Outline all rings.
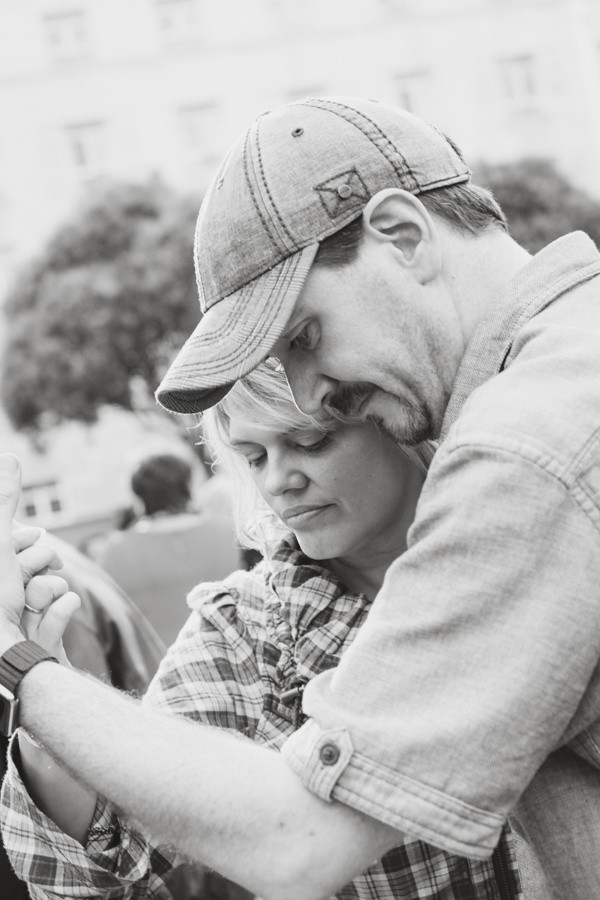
[25,603,43,614]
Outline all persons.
[0,94,600,900]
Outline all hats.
[133,454,192,508]
[153,95,470,411]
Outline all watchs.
[0,640,59,737]
